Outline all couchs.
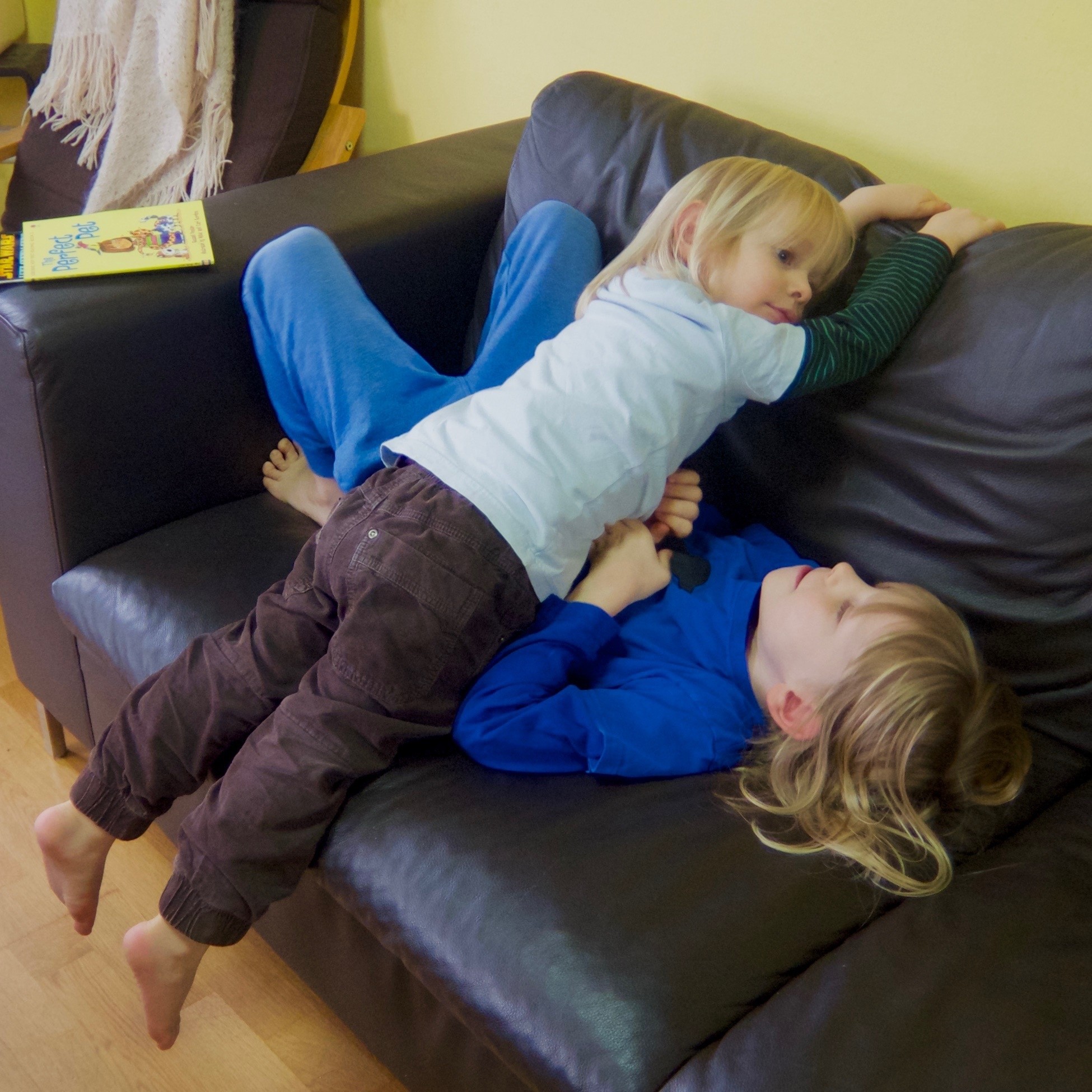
[0,69,1092,1092]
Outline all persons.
[36,160,1008,1048]
[243,200,1033,900]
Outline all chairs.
[0,0,366,233]
